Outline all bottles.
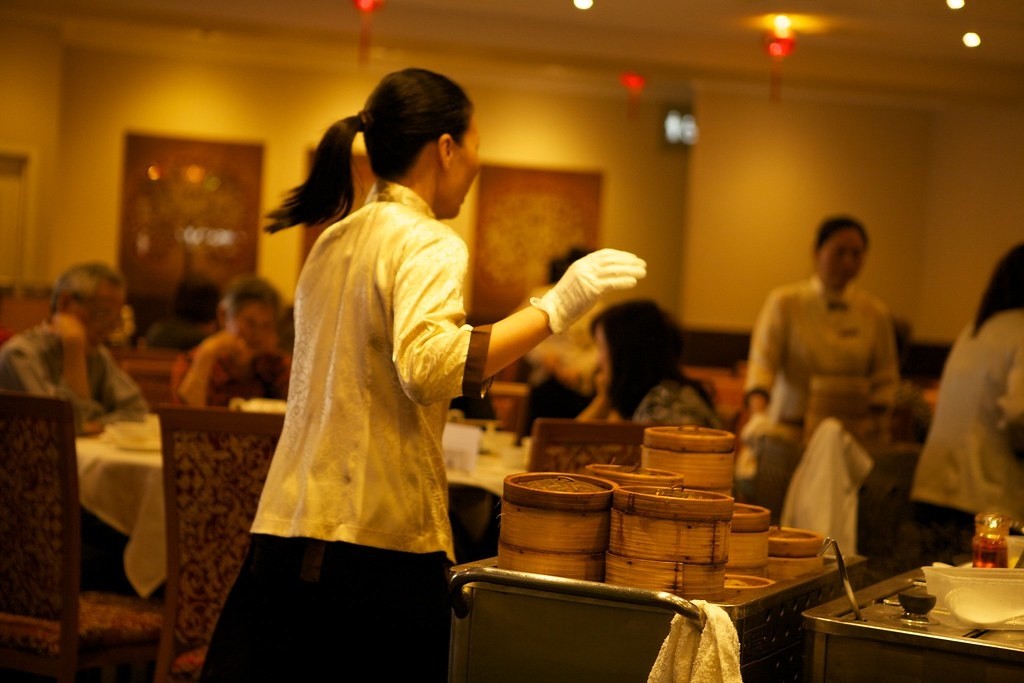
[972,512,1011,568]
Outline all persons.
[193,68,646,683]
[578,297,720,429]
[506,245,608,429]
[0,261,149,439]
[906,242,1024,561]
[174,272,292,408]
[746,217,898,442]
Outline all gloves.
[530,248,646,335]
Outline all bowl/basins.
[441,421,479,480]
[921,564,1024,631]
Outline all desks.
[77,433,529,600]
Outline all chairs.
[0,349,663,683]
